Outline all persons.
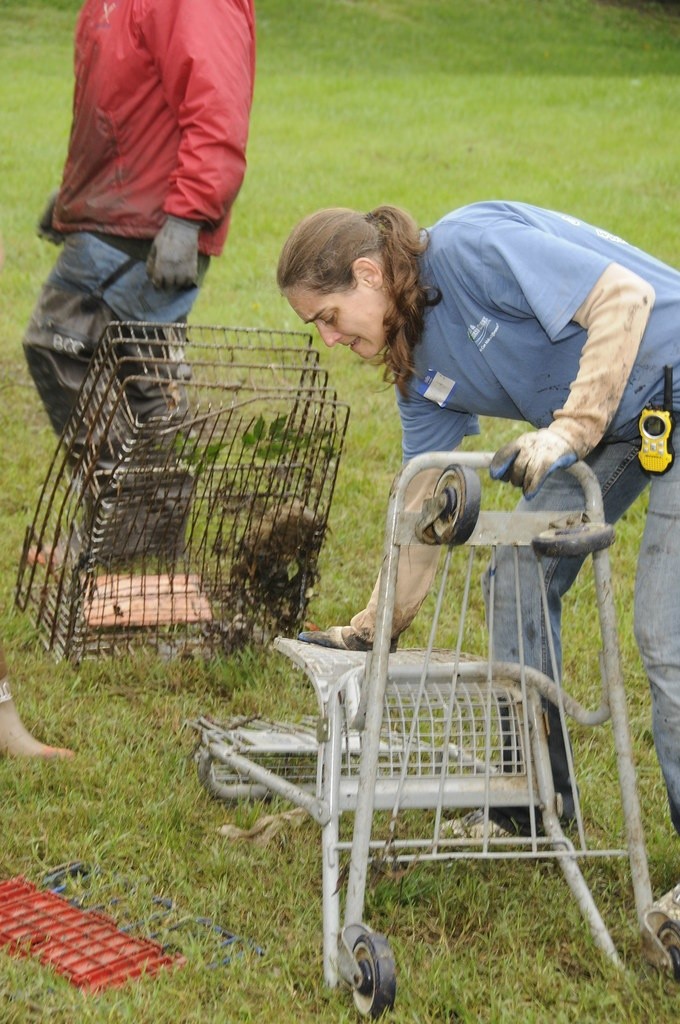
[21,0,257,557]
[276,199,680,920]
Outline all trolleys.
[186,451,680,1022]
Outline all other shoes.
[439,810,513,837]
[654,883,680,919]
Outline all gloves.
[488,428,578,500]
[145,215,204,291]
[298,627,351,651]
[37,193,63,244]
